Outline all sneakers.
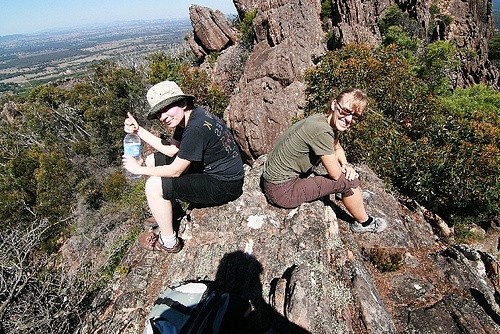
[350,215,388,233]
[337,192,369,200]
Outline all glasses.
[337,102,360,120]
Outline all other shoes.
[139,233,181,252]
[145,215,156,226]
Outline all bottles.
[123,126,141,179]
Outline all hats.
[146,81,196,119]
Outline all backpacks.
[140,279,221,334]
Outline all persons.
[261,88,386,234]
[121,81,243,253]
